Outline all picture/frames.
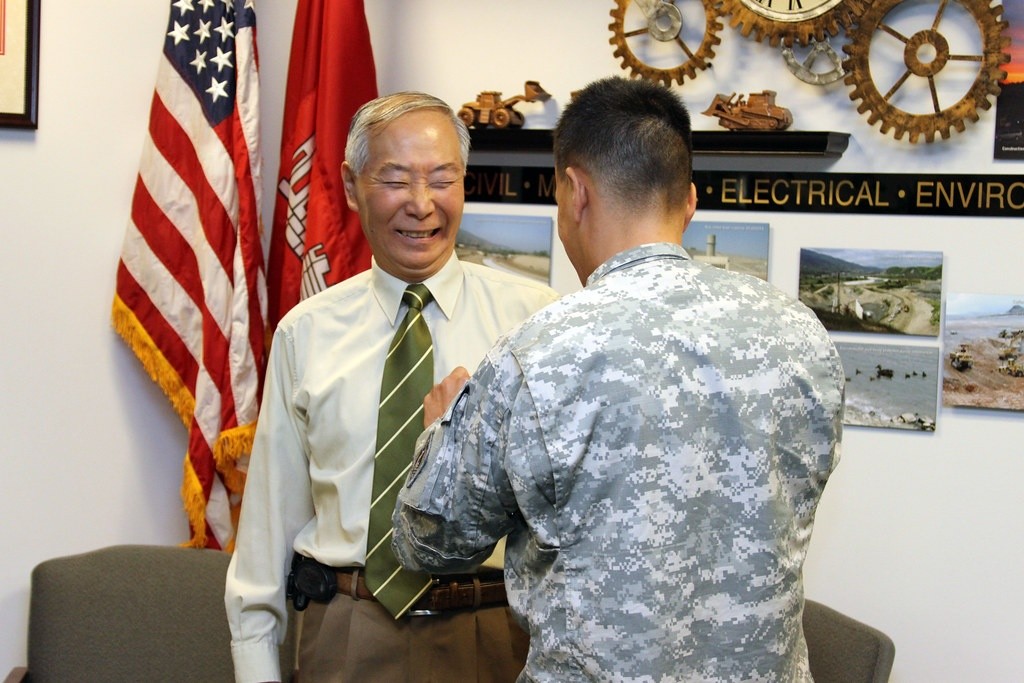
[0,0,41,131]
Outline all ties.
[364,283,433,617]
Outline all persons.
[224,90,561,683]
[389,76,847,683]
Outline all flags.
[111,0,273,552]
[265,0,378,334]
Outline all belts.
[335,573,508,618]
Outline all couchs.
[3,542,896,683]
